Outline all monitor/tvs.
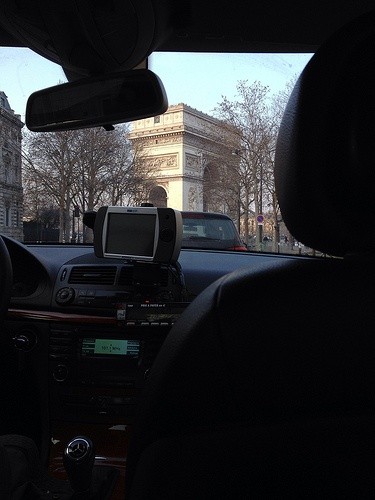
[94,206,183,264]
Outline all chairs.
[126,38,374,499]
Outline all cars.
[179,209,249,252]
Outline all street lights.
[231,148,276,251]
[51,141,120,243]
